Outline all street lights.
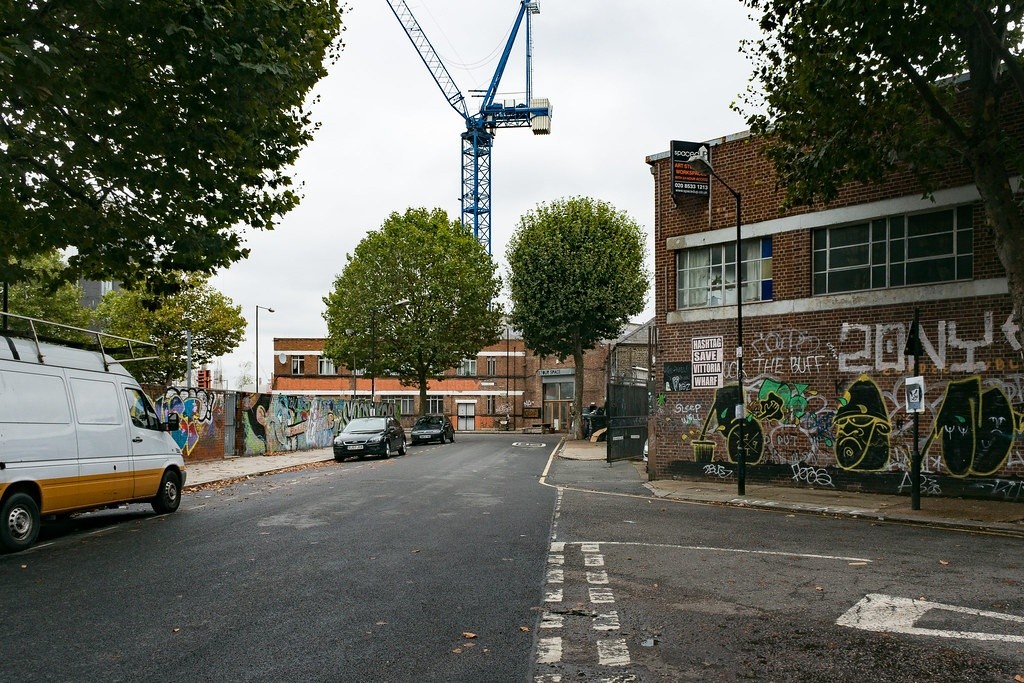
[255,305,275,394]
[686,154,748,500]
[502,324,510,423]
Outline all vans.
[0,312,187,551]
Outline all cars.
[333,417,407,462]
[410,413,455,445]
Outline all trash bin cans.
[580,413,590,439]
[588,414,605,441]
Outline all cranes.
[389,0,552,260]
